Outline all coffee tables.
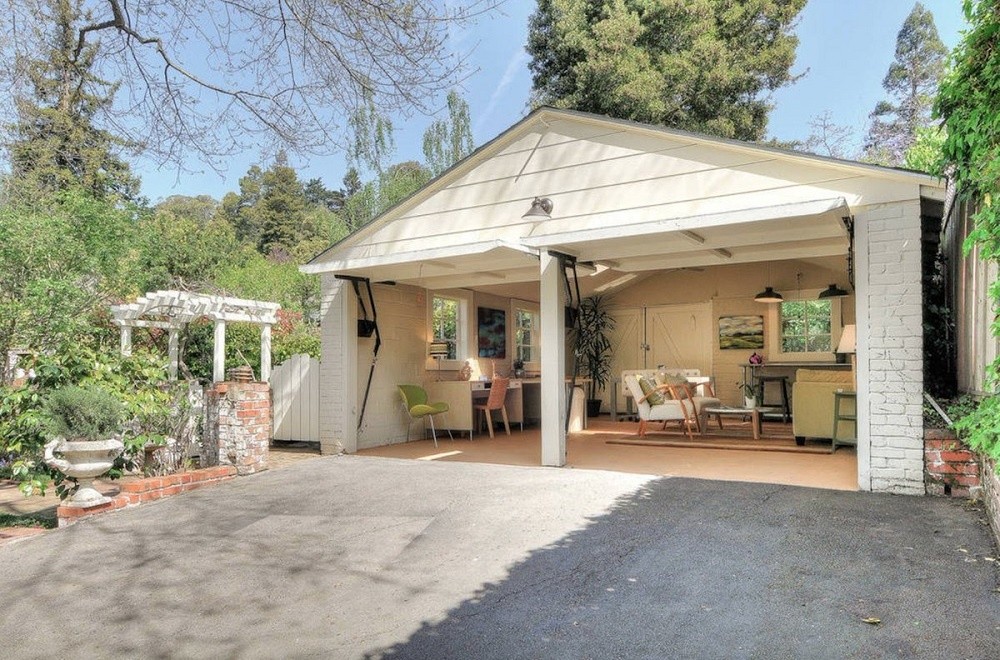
[831,391,857,454]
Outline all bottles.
[461,361,470,380]
[749,351,762,364]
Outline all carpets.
[604,424,841,454]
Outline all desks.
[738,362,853,422]
[423,377,593,442]
[700,406,772,440]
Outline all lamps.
[521,196,553,221]
[836,325,857,391]
[429,343,449,382]
[754,262,785,303]
[817,257,848,298]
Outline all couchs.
[792,368,853,445]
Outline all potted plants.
[566,294,617,417]
[36,381,128,510]
[513,359,524,378]
[738,382,763,408]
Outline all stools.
[753,375,791,425]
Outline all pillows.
[639,373,694,407]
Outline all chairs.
[471,376,510,439]
[396,384,454,448]
[624,372,723,442]
